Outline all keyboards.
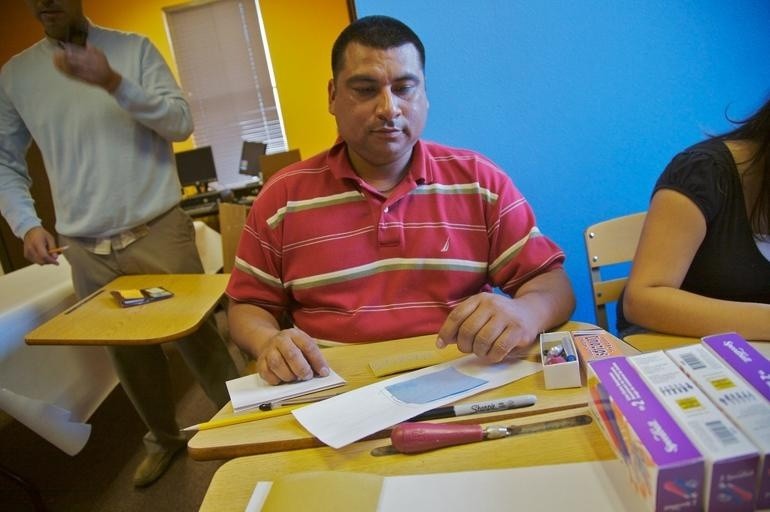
[183,201,216,214]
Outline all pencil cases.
[573,332,624,378]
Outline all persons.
[223,13,578,387]
[0,0,239,489]
[620,97,770,342]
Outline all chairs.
[0,142,304,512]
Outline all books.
[224,363,347,414]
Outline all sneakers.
[132,426,188,487]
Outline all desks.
[187,211,766,511]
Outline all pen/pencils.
[405,395,537,423]
[47,245,69,254]
[179,392,345,432]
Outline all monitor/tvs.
[174,145,218,194]
[240,141,267,187]
